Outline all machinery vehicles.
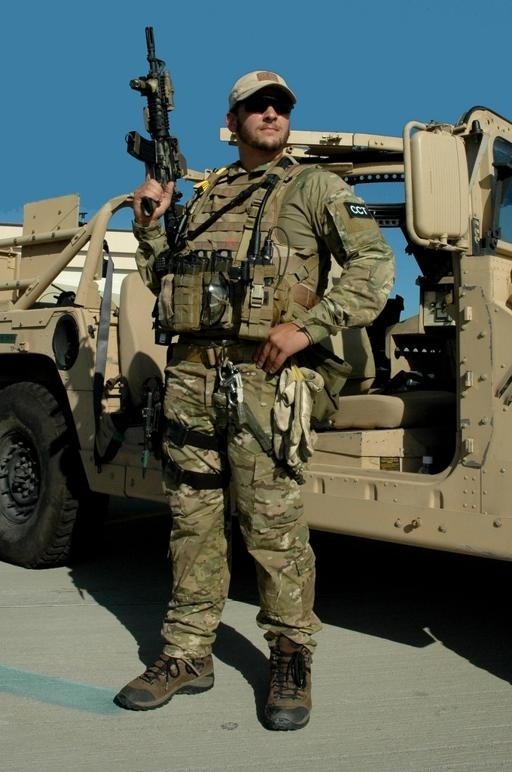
[1,106,511,678]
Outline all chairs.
[116,271,172,415]
[319,270,457,430]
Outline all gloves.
[272,366,324,468]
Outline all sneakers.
[112,651,215,713]
[262,635,314,731]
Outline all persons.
[114,70,394,731]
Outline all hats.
[227,71,299,111]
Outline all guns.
[141,389,153,478]
[127,25,181,252]
